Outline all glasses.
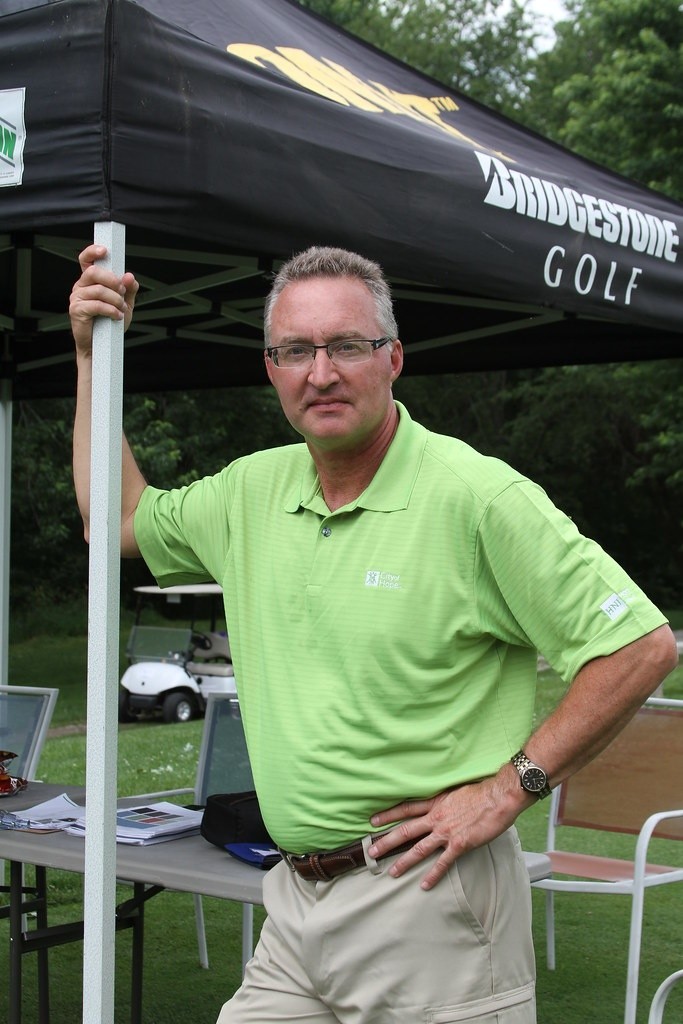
[263,337,390,369]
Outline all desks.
[0,797,552,1024]
[0,782,86,1024]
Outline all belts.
[285,827,435,882]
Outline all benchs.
[185,635,234,677]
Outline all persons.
[62,245,676,1024]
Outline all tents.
[0,1,683,1024]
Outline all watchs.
[510,751,552,801]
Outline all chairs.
[0,685,59,933]
[116,691,255,983]
[530,698,683,1024]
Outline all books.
[65,800,204,846]
